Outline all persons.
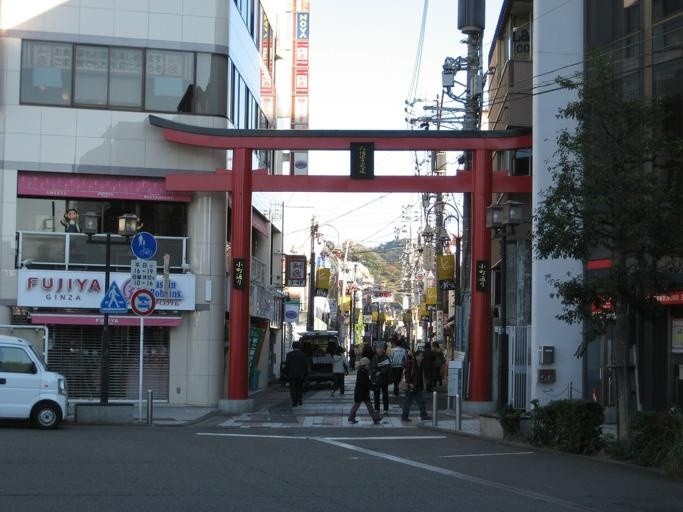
[286,342,306,406]
[332,351,349,394]
[346,334,449,424]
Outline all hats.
[360,357,370,365]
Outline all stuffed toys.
[60,209,80,233]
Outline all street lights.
[422,201,464,351]
[280,291,291,364]
[82,212,144,406]
[348,283,361,349]
[486,201,527,418]
[309,225,343,331]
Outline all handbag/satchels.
[398,375,408,391]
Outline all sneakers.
[292,401,302,406]
[421,416,432,420]
[374,410,388,423]
[348,421,358,424]
[402,419,412,422]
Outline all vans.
[0,335,69,430]
[296,330,342,388]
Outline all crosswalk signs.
[100,280,128,315]
